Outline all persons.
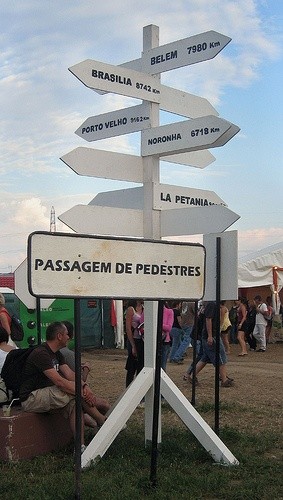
[0,290,282,429]
[22,321,129,455]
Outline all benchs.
[0,405,66,462]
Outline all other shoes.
[188,377,201,387]
[221,378,235,388]
[183,375,192,381]
[249,348,256,351]
[255,348,266,352]
[219,376,233,381]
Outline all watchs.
[82,383,88,389]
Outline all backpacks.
[2,311,25,342]
[259,302,273,320]
[0,344,38,400]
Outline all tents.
[238,242,283,315]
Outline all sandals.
[238,352,248,357]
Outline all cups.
[3,405,11,417]
